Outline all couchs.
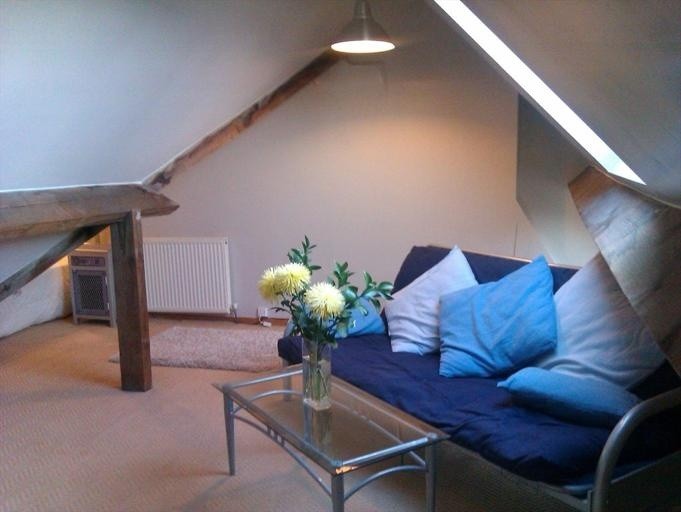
[275,245,681,511]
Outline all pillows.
[438,254,558,379]
[534,251,665,392]
[494,365,662,425]
[385,245,480,355]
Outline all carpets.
[105,324,294,374]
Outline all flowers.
[256,235,398,396]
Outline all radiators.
[140,235,238,317]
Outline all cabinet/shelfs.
[68,245,114,325]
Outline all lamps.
[329,0,397,55]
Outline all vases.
[300,335,334,411]
